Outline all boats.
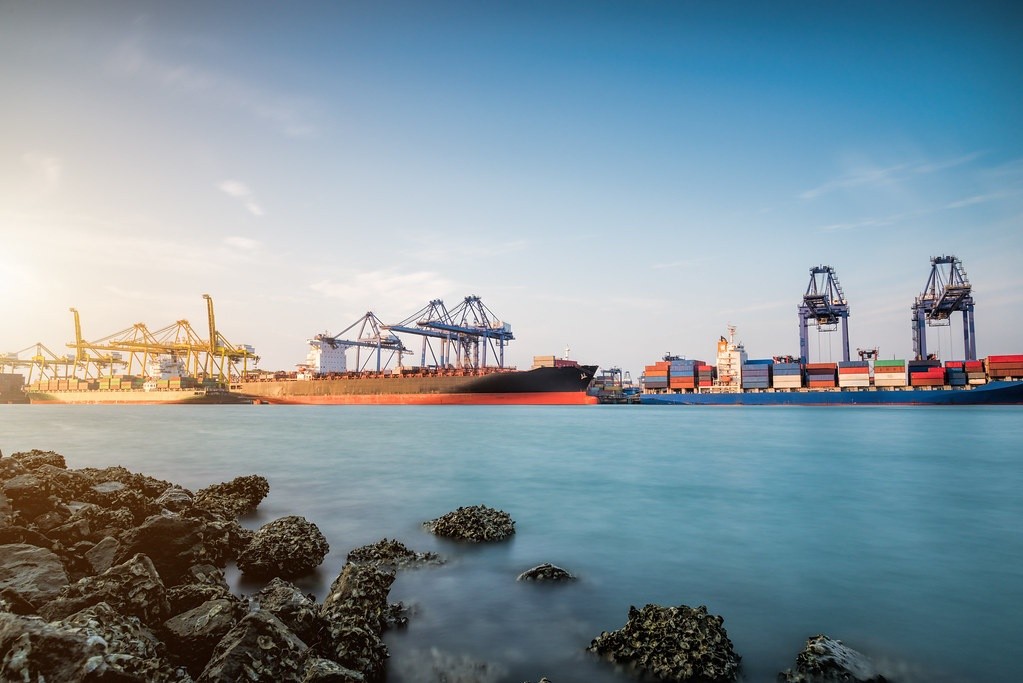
[587,347,1023,404]
[230,364,601,405]
[0,351,239,405]
